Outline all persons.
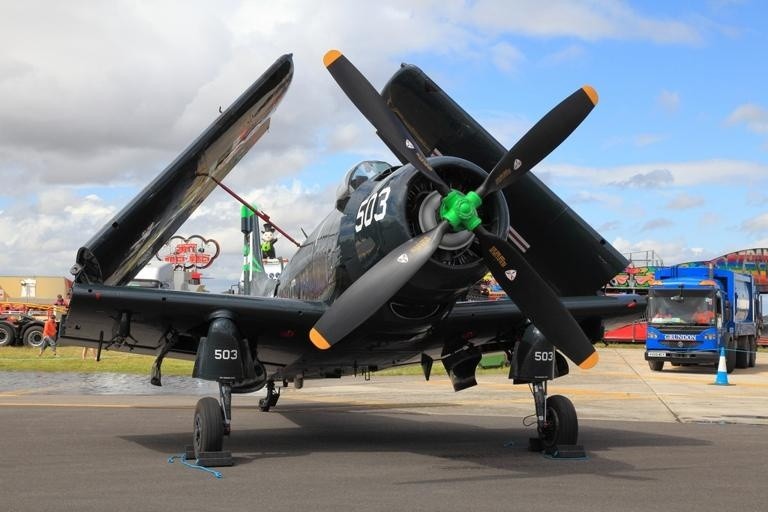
[691,303,714,325]
[654,305,672,318]
[38,314,58,357]
[27,307,33,319]
[54,295,65,306]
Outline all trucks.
[645,267,758,374]
[0,302,69,348]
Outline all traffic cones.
[715,347,729,385]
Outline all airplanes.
[59,49,648,459]
